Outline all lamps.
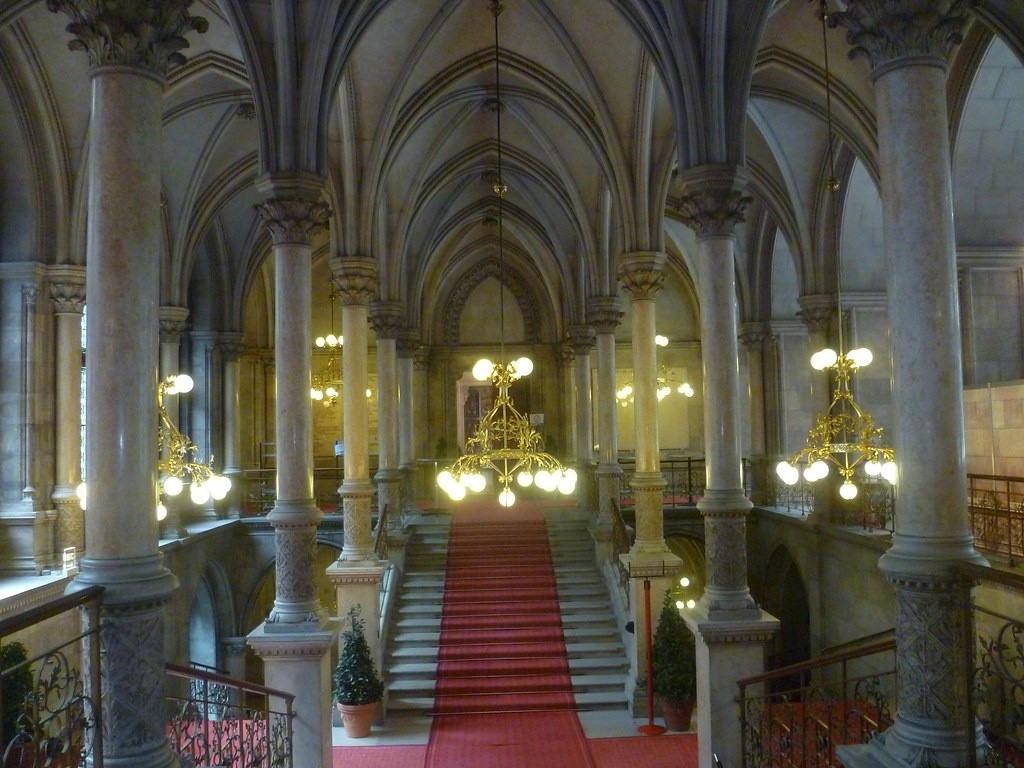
[617,334,694,403]
[437,0,579,507]
[310,272,372,407]
[76,374,231,522]
[775,0,899,500]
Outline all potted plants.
[0,641,44,768]
[635,587,698,731]
[333,604,385,738]
